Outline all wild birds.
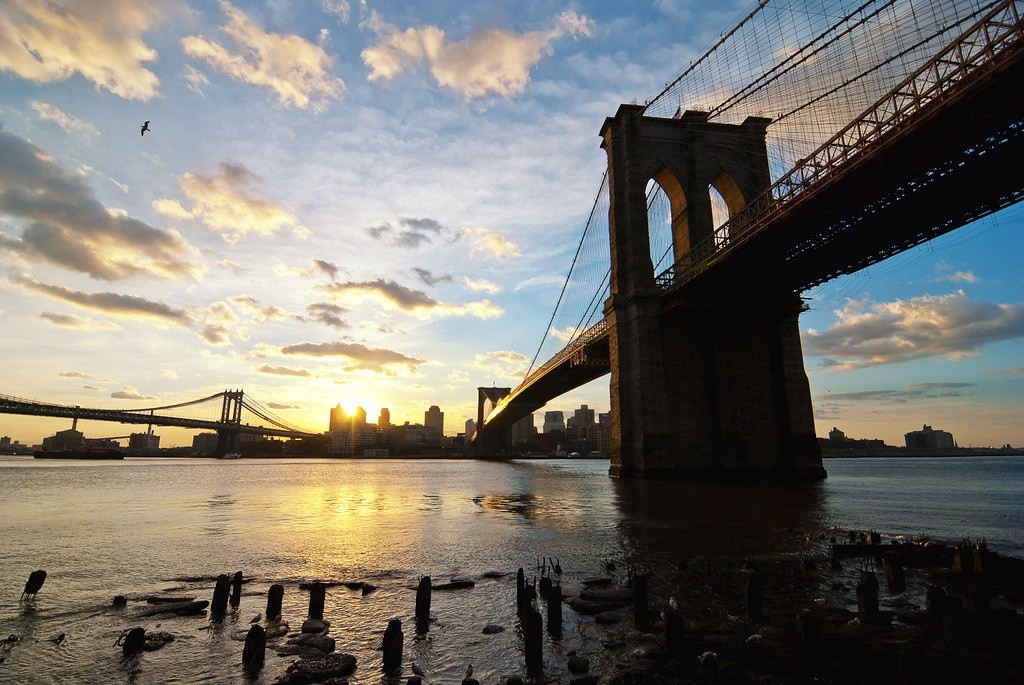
[465,664,475,680]
[669,597,679,609]
[412,661,427,678]
[44,633,65,646]
[140,121,150,136]
[247,613,263,626]
